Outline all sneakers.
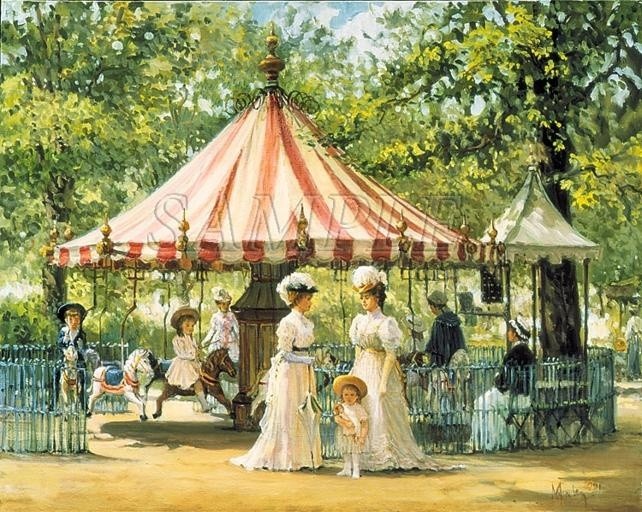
[336,470,352,477]
[351,469,360,480]
[201,404,216,414]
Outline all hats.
[333,374,368,397]
[406,314,427,333]
[508,312,532,342]
[213,290,232,303]
[171,308,200,329]
[57,303,86,323]
[276,272,320,305]
[426,290,448,306]
[351,266,388,294]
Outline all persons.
[470,313,537,451]
[47,299,96,418]
[331,375,370,478]
[200,287,240,418]
[163,304,217,414]
[228,267,326,472]
[619,304,642,378]
[333,404,359,443]
[424,288,467,369]
[349,263,467,473]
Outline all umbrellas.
[483,166,601,360]
